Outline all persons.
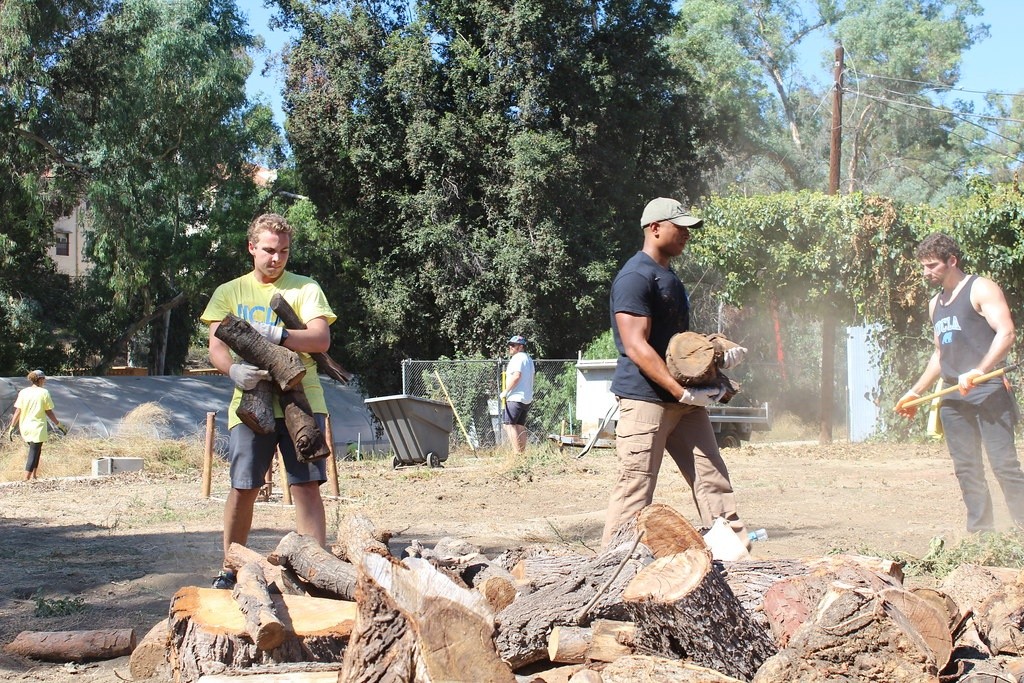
[599,196,748,564]
[893,234,1024,534]
[8,370,68,482]
[499,335,536,454]
[199,214,338,592]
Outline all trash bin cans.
[364,394,453,470]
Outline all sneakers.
[211,570,237,590]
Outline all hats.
[507,336,525,345]
[33,370,45,381]
[641,197,704,229]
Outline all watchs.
[278,328,289,347]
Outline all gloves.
[500,389,509,400]
[720,347,748,369]
[56,421,68,435]
[249,321,283,345]
[679,383,726,406]
[229,364,262,390]
[8,426,15,442]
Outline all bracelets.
[907,390,921,400]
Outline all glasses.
[510,344,518,347]
